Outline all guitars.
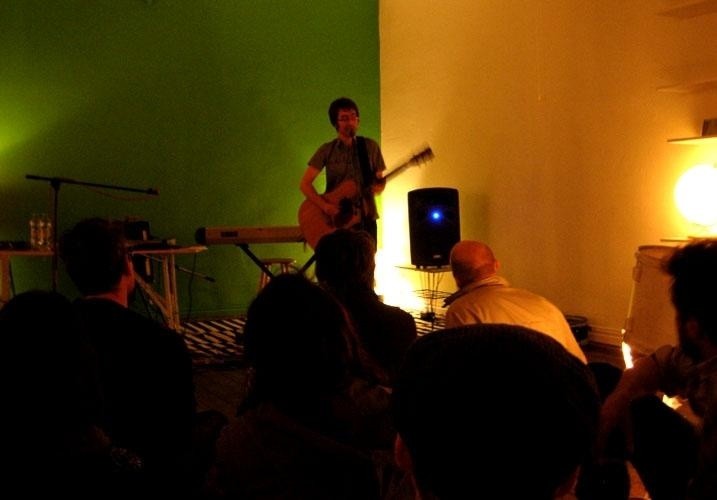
[298,144,435,249]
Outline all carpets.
[179,313,444,370]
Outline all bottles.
[29,211,54,252]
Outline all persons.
[224,227,716,499]
[2,218,222,499]
[300,98,386,290]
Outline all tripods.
[128,246,216,328]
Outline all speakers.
[408,187,460,269]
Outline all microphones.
[349,128,358,154]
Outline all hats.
[397,324,599,492]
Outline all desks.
[397,264,454,321]
[1,240,206,334]
